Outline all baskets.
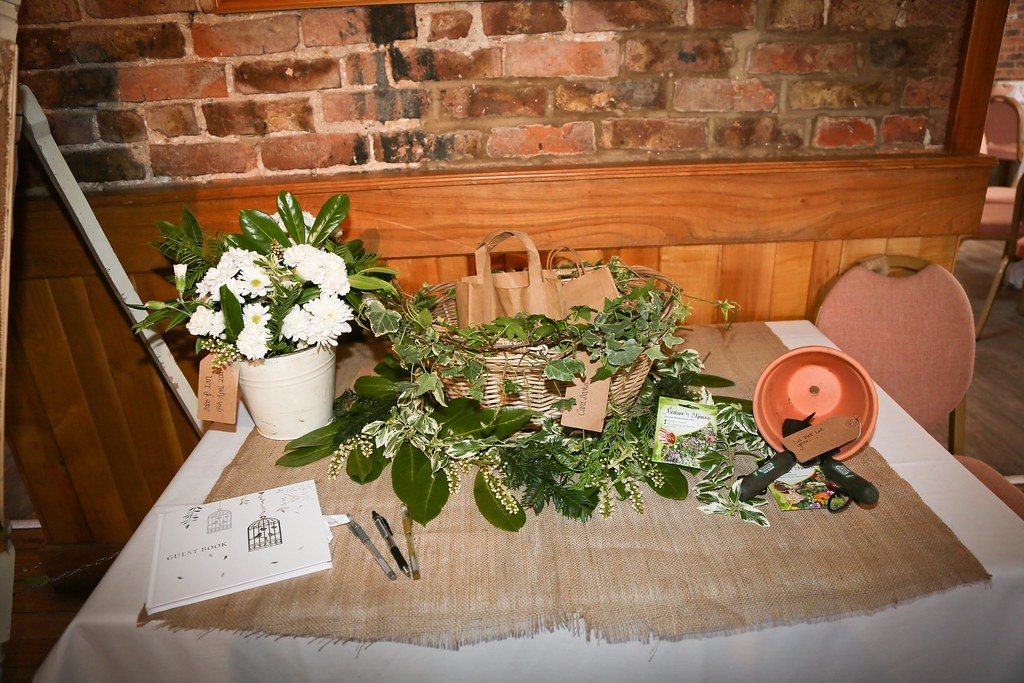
[402,268,681,426]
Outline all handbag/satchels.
[455,231,624,338]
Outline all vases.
[234,340,338,443]
[751,348,878,462]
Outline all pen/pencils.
[372,510,411,578]
[401,503,421,580]
[346,514,398,580]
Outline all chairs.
[815,94,1024,521]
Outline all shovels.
[738,411,881,507]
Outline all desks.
[29,325,1024,683]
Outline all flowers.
[119,189,404,374]
[274,255,771,531]
